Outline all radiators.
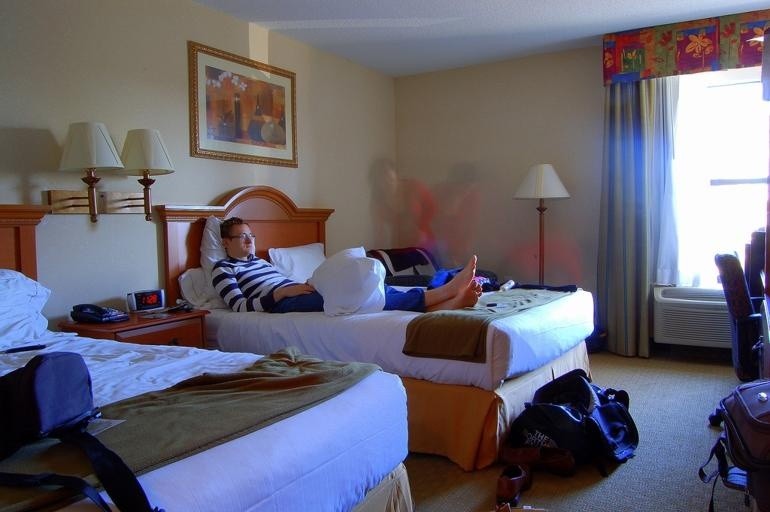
[653,283,732,349]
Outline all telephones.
[70,304,128,323]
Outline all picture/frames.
[187,39,299,169]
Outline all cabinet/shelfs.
[758,270,770,380]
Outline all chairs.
[715,253,764,383]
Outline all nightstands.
[56,307,212,350]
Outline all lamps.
[48,122,175,223]
[512,163,572,286]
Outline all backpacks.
[428,267,500,293]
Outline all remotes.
[7,344,46,353]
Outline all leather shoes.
[496,462,538,506]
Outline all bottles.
[498,280,516,292]
[234,93,285,143]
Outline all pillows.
[177,266,228,311]
[199,215,256,300]
[307,246,387,316]
[267,242,326,283]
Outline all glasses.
[223,233,255,240]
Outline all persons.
[209,215,482,314]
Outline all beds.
[154,185,595,473]
[0,202,417,512]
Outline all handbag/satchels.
[503,368,639,470]
[1,350,164,512]
[719,377,770,472]
